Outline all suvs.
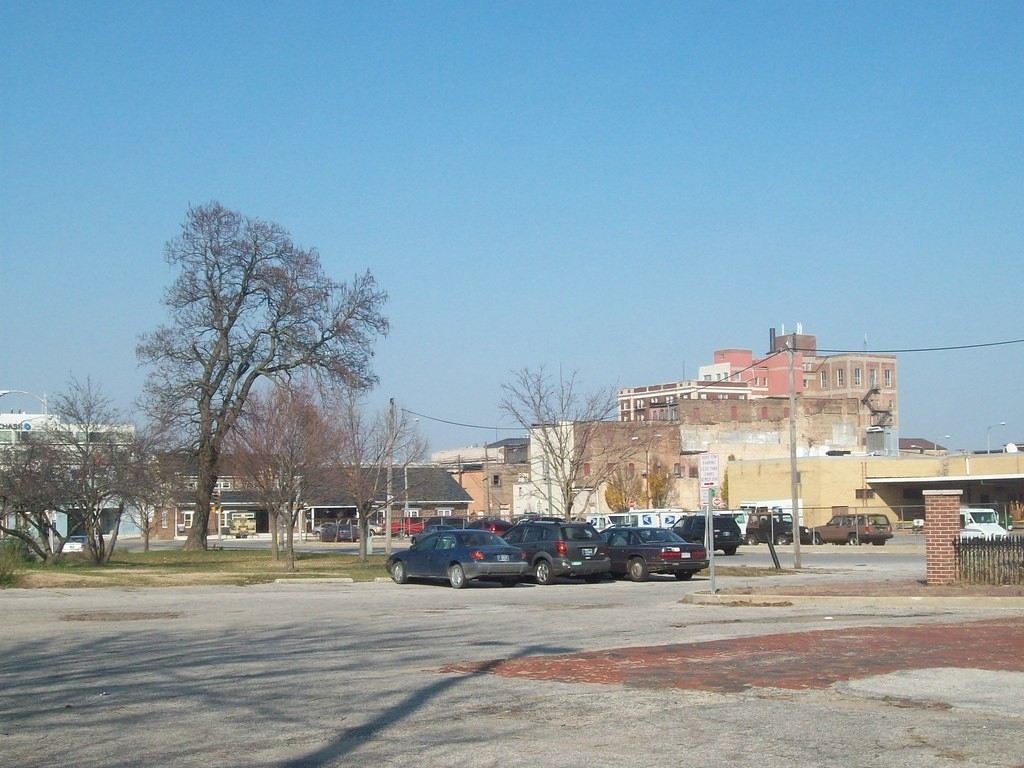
[962,508,1014,540]
[746,512,809,545]
[667,516,743,556]
[502,518,612,586]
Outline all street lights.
[934,436,949,456]
[0,390,49,430]
[987,422,1006,454]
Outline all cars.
[599,524,710,582]
[339,517,384,538]
[312,523,335,541]
[237,531,259,538]
[321,524,357,542]
[384,516,428,537]
[63,536,99,553]
[385,530,529,589]
[467,520,513,537]
[809,514,893,545]
[410,524,457,545]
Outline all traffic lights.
[214,494,220,505]
